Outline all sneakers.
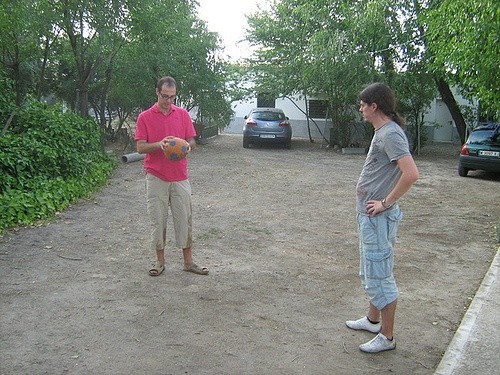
[345,315,382,334]
[358,332,397,353]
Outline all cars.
[457,122,500,178]
[241,108,293,148]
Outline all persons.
[344,82,420,353]
[134,76,210,276]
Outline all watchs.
[380,197,392,209]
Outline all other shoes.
[149,262,164,276]
[182,262,209,275]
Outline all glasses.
[159,91,177,99]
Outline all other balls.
[164,137,188,160]
